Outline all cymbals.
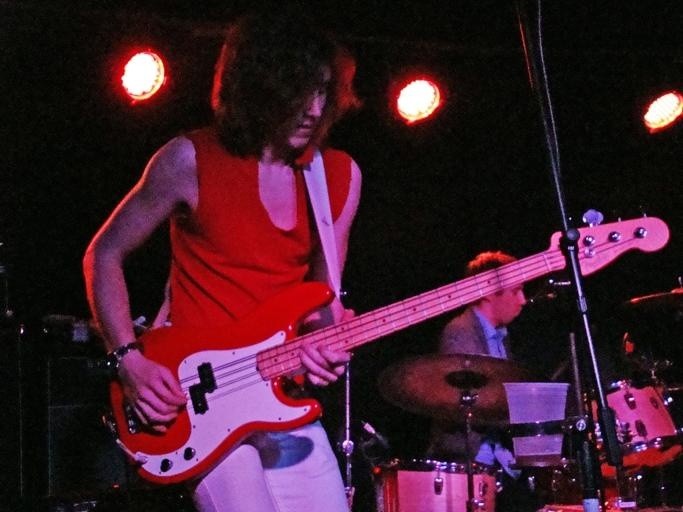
[374,351,540,419]
[620,288,682,306]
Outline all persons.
[426,251,528,464]
[83,12,362,512]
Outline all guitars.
[108,206,670,485]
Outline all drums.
[368,460,502,510]
[581,376,682,479]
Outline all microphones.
[362,423,390,449]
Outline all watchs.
[105,341,144,373]
